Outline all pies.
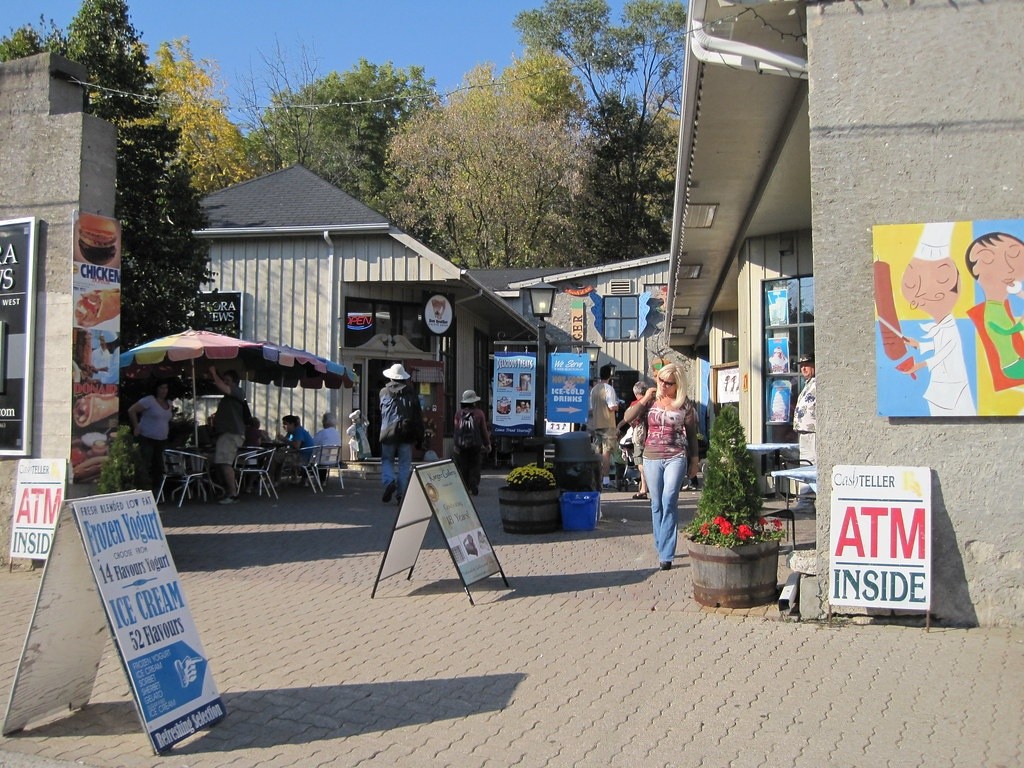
[74,287,121,327]
[74,394,119,427]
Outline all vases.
[498,486,560,535]
[685,538,779,609]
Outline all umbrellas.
[119,324,266,447]
[234,337,362,437]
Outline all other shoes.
[633,492,648,499]
[215,491,240,505]
[321,481,326,487]
[395,495,404,506]
[659,561,672,570]
[471,484,479,496]
[302,481,309,488]
[381,481,398,503]
[602,483,618,491]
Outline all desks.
[176,447,215,497]
[771,465,817,542]
[260,443,288,460]
[744,443,800,500]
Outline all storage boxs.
[558,491,601,531]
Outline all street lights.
[522,275,559,475]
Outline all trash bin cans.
[554,431,602,520]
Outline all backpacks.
[454,408,483,450]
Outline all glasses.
[658,376,676,386]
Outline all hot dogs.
[73,457,111,483]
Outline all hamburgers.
[78,215,117,248]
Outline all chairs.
[758,455,813,550]
[156,444,344,507]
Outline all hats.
[459,390,481,404]
[382,363,411,380]
[796,353,814,364]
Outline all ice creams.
[771,391,786,422]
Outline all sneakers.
[788,502,816,514]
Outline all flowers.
[505,462,556,494]
[679,402,790,549]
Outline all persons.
[309,411,342,488]
[453,389,492,496]
[127,376,174,506]
[589,364,625,491]
[624,363,700,572]
[617,381,655,500]
[203,364,271,506]
[73,327,119,385]
[346,409,372,463]
[380,363,424,506]
[259,414,315,488]
[789,353,818,513]
